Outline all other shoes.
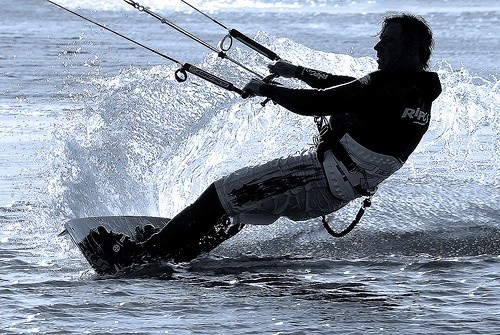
[137,223,172,256]
[93,226,141,265]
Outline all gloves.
[241,78,274,100]
[268,62,298,79]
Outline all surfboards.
[58,215,172,275]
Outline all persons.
[88,10,446,269]
